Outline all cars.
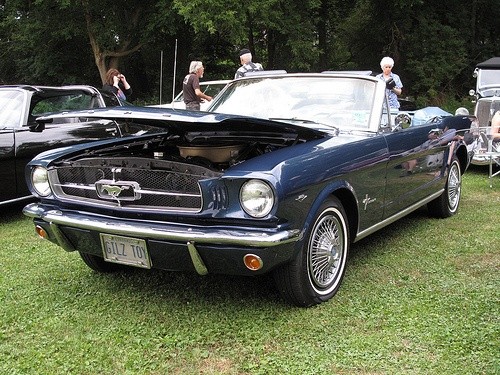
[455,56,500,166]
[0,82,142,213]
[143,79,236,115]
[20,71,481,307]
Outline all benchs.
[31,110,81,123]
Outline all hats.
[238,49,251,56]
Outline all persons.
[489,110,500,152]
[234,49,264,79]
[100,68,133,104]
[183,61,212,111]
[374,56,403,109]
[455,106,484,154]
